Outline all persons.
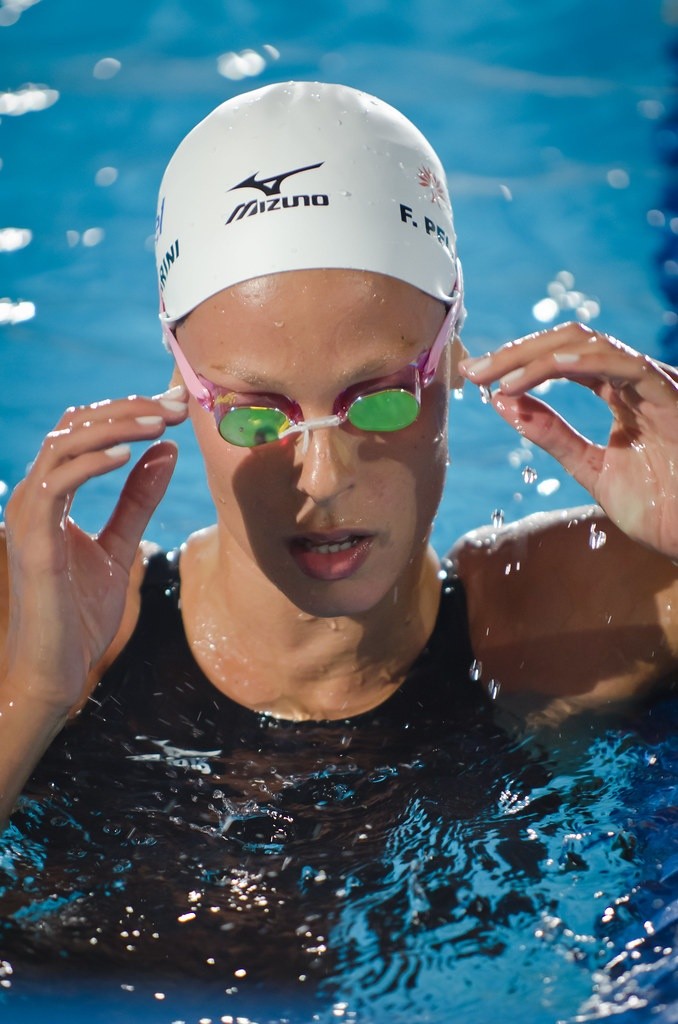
[0,79,678,818]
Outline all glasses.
[195,362,421,447]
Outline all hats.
[152,81,460,331]
